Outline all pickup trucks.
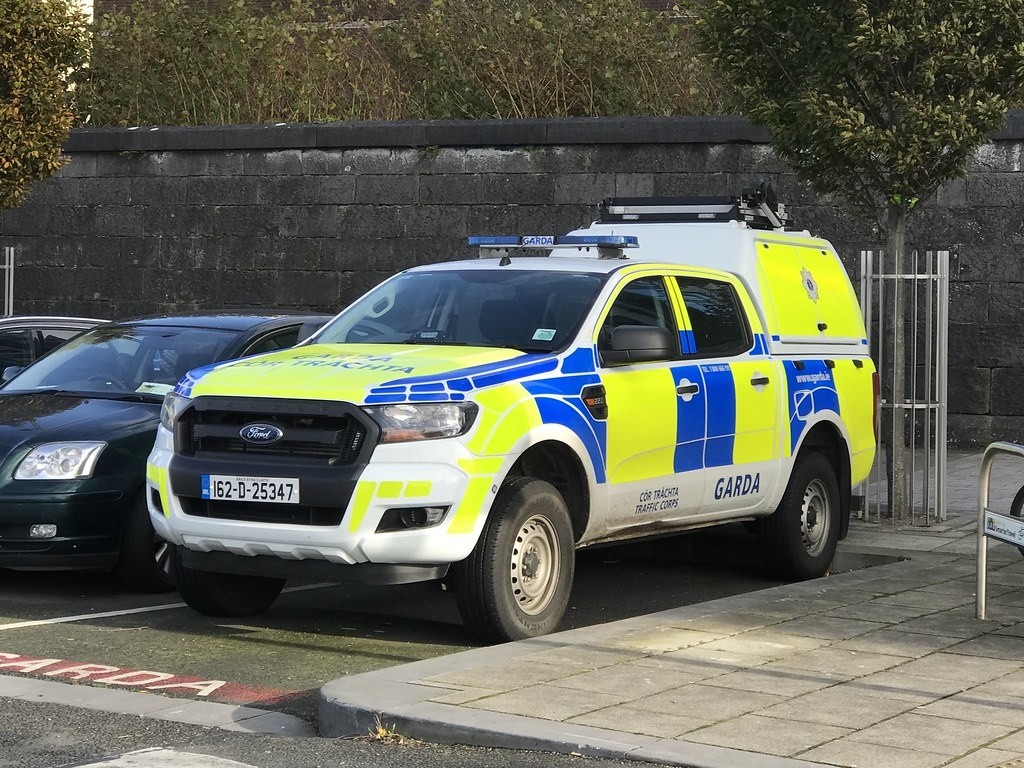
[148,193,884,642]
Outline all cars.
[1,312,333,591]
[1,317,144,404]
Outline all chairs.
[541,288,609,352]
[479,299,531,347]
